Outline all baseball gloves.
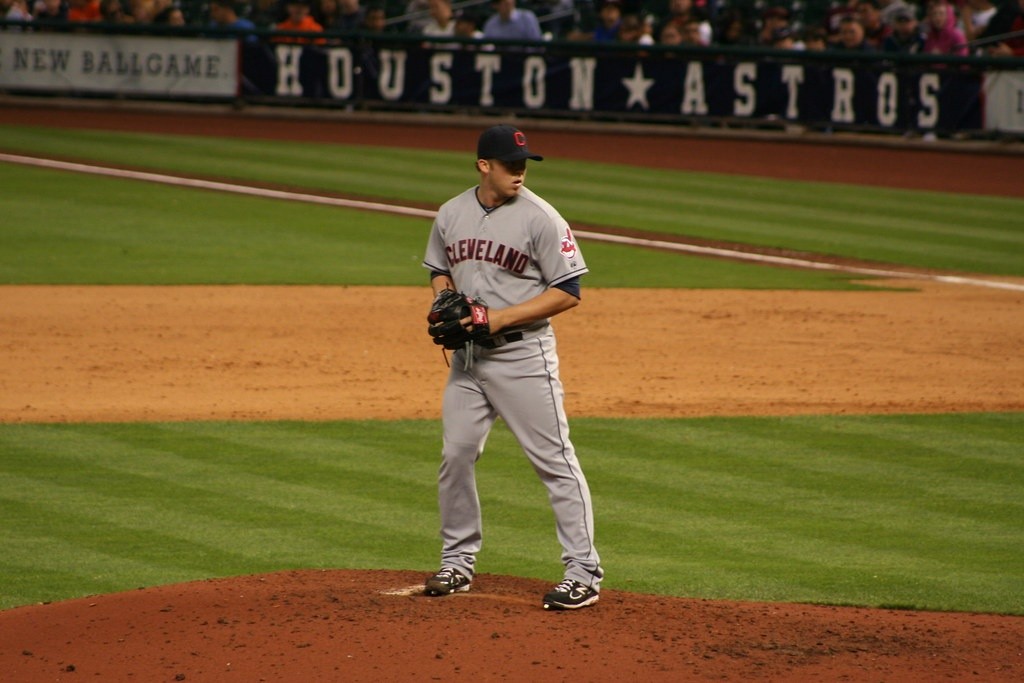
[427,281,491,369]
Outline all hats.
[477,124,544,162]
[894,8,915,20]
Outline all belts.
[473,330,522,350]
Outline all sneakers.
[543,578,599,608]
[427,567,471,593]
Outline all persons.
[422,124,604,610]
[0,0,1024,144]
